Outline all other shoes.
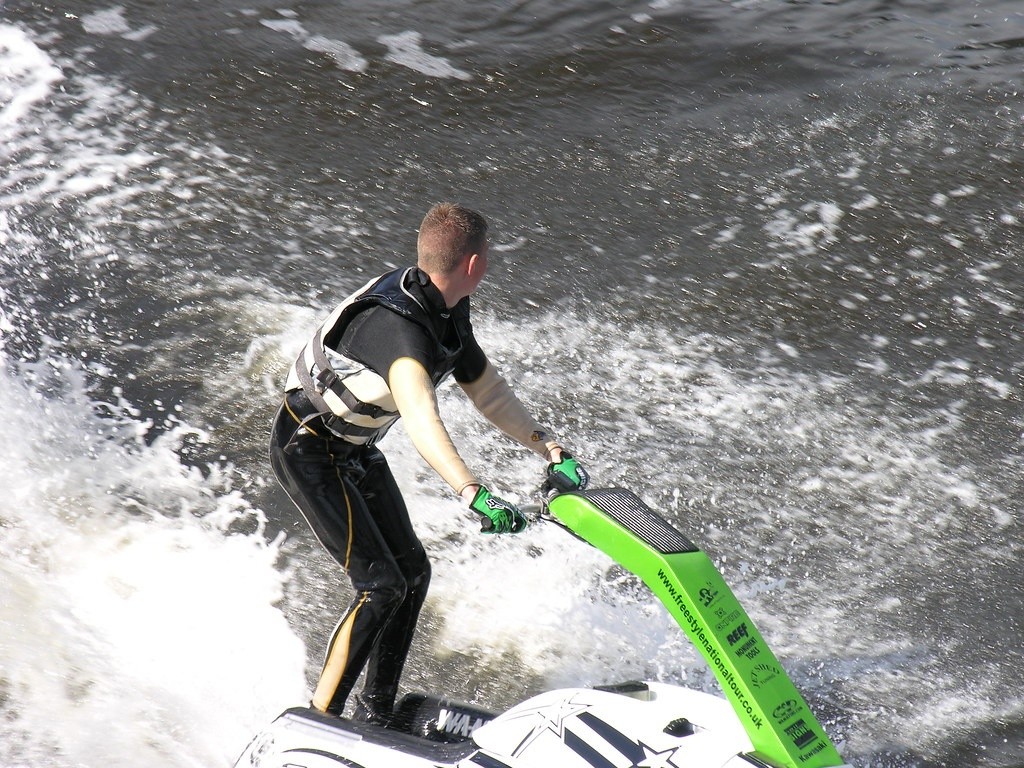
[352,705,410,734]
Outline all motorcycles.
[226,479,852,767]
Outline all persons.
[269,202,591,729]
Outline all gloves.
[469,487,531,534]
[541,451,590,493]
